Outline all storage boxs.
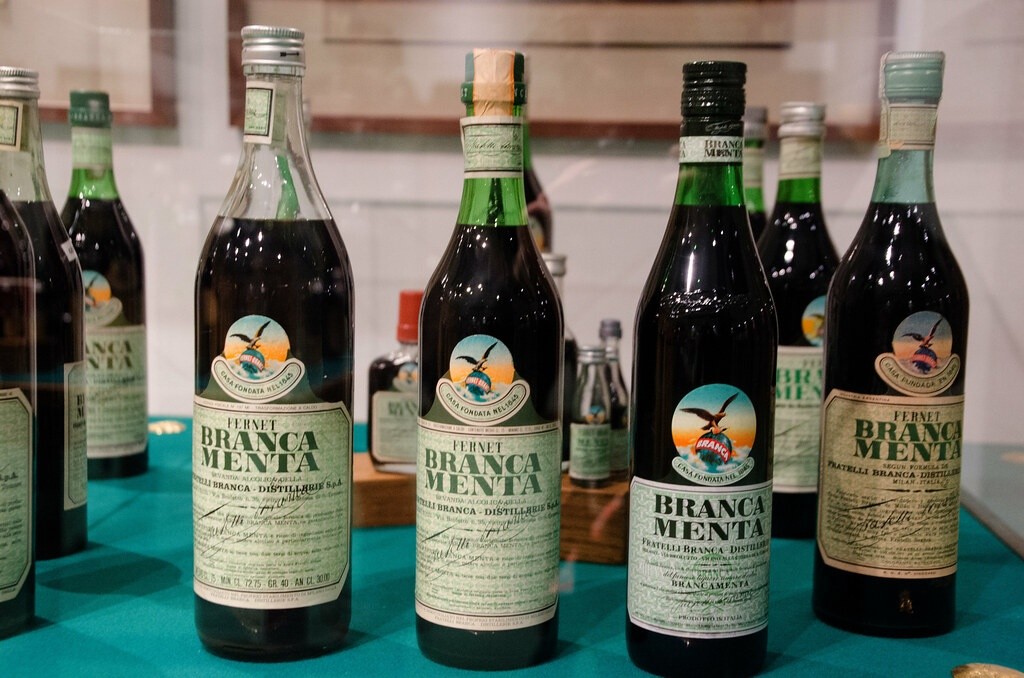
[555,473,630,564]
[350,451,416,528]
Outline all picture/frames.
[0,0,177,127]
[228,1,895,139]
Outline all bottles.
[0,23,970,678]
